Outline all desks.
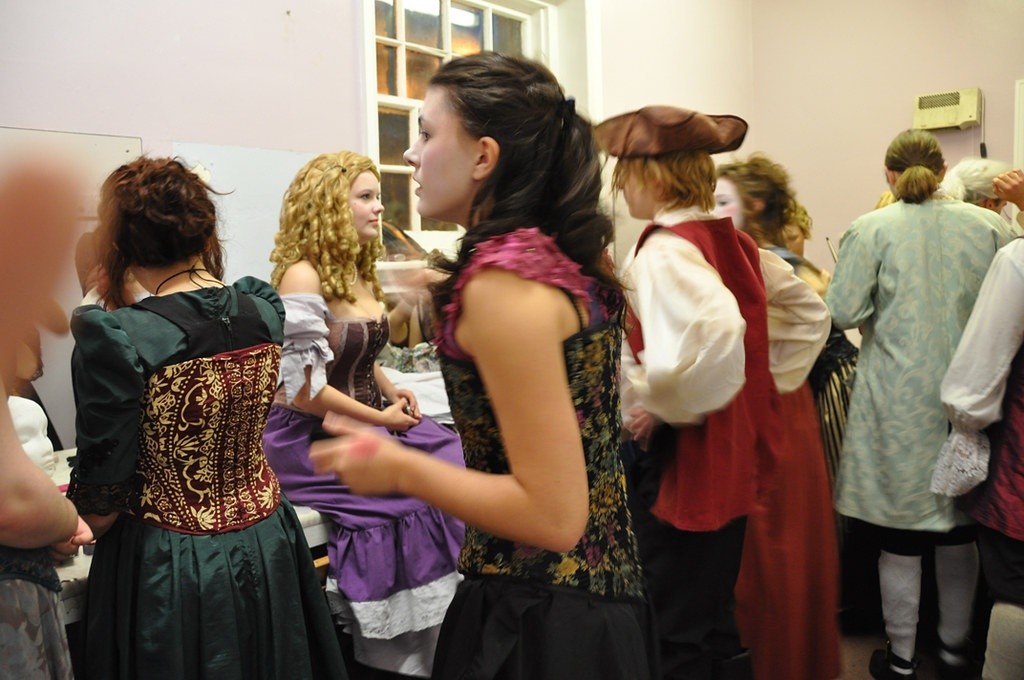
[51,370,452,625]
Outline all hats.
[592,106,749,159]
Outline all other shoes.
[869,640,921,680]
[935,635,980,680]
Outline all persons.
[312,47,653,679]
[0,107,1024,680]
